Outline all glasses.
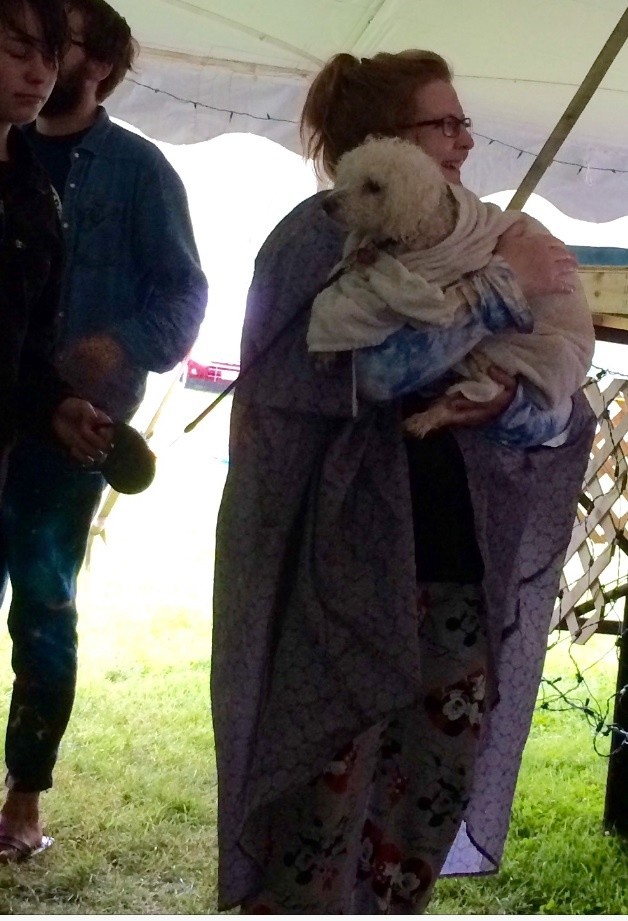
[59,35,86,61]
[399,116,471,138]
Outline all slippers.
[0,836,55,864]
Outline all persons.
[212,49,597,915]
[0,0,208,865]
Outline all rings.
[108,443,114,448]
[96,450,104,458]
[84,456,95,466]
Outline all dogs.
[319,135,523,434]
